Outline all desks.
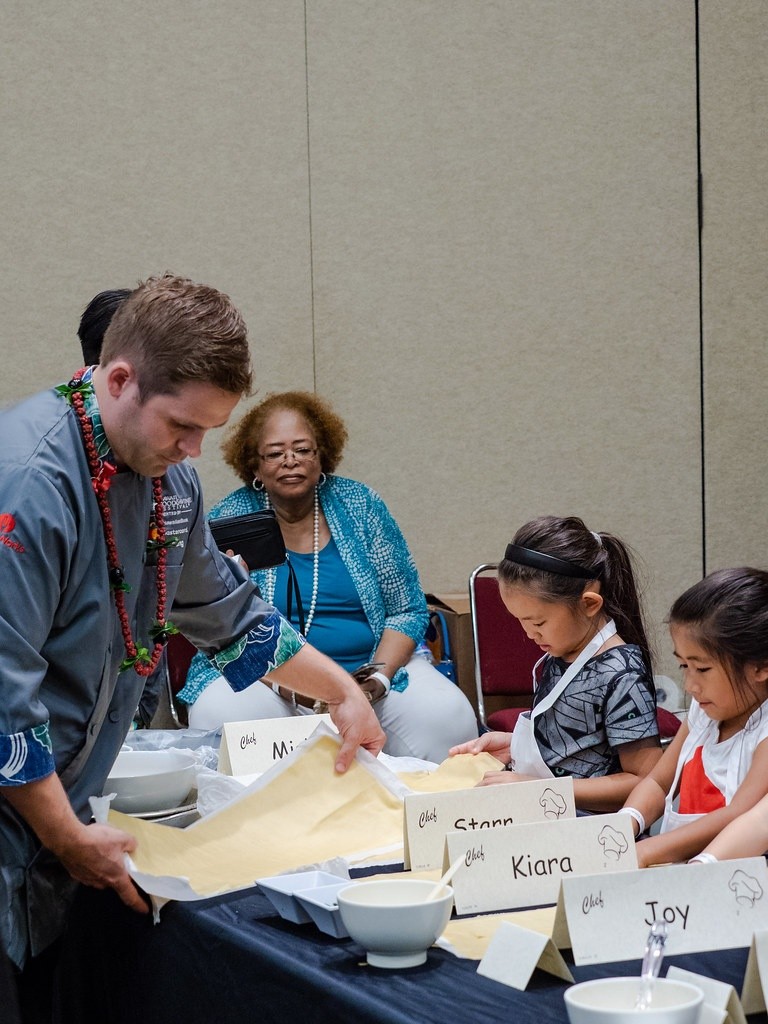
[40,728,768,1024]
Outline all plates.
[94,789,200,831]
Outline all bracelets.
[360,668,391,705]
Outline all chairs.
[468,563,684,748]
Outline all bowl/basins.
[336,880,453,970]
[563,974,703,1024]
[105,749,198,812]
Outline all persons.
[177,393,479,774]
[0,275,384,1019]
[449,515,664,812]
[610,568,768,869]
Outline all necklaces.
[260,487,320,657]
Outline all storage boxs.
[423,590,539,730]
